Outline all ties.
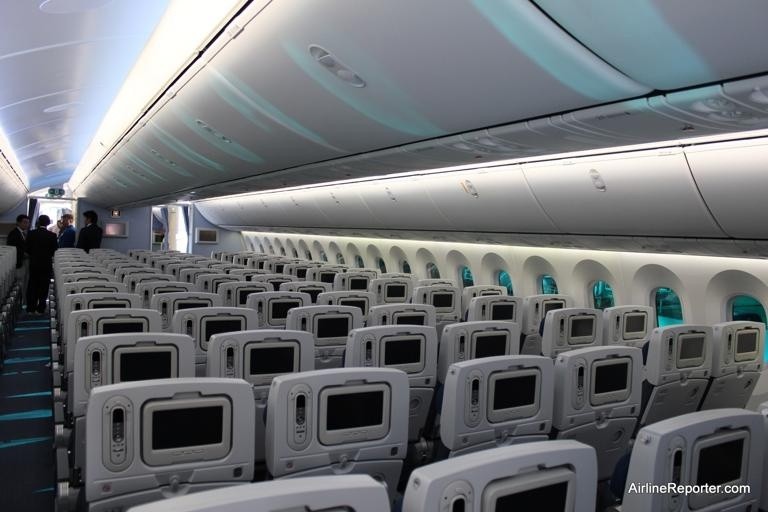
[22,232,26,240]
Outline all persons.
[57,214,75,249]
[5,213,30,307]
[25,215,58,317]
[76,210,103,253]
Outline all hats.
[57,208,62,221]
[62,208,72,216]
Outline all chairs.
[0,245,24,352]
[50,247,768,512]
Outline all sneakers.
[27,311,43,316]
